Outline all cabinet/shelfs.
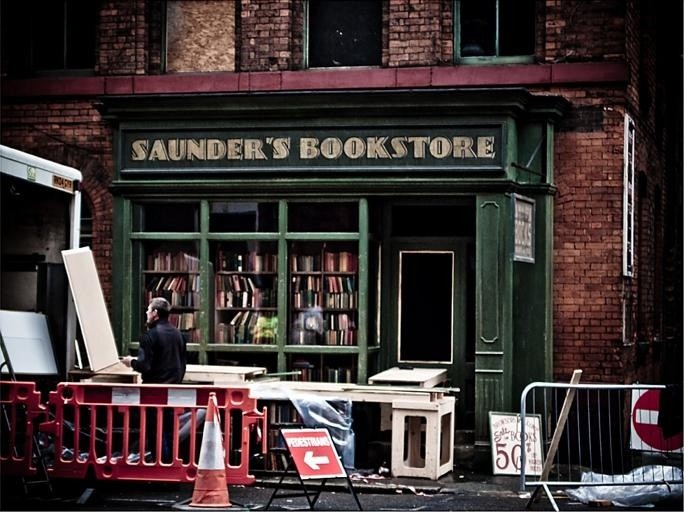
[143,267,199,313]
[290,269,358,313]
[214,269,279,312]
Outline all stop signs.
[627,386,683,455]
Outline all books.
[146,251,359,383]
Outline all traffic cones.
[172,390,247,510]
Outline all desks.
[221,375,462,479]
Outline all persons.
[121,297,186,492]
